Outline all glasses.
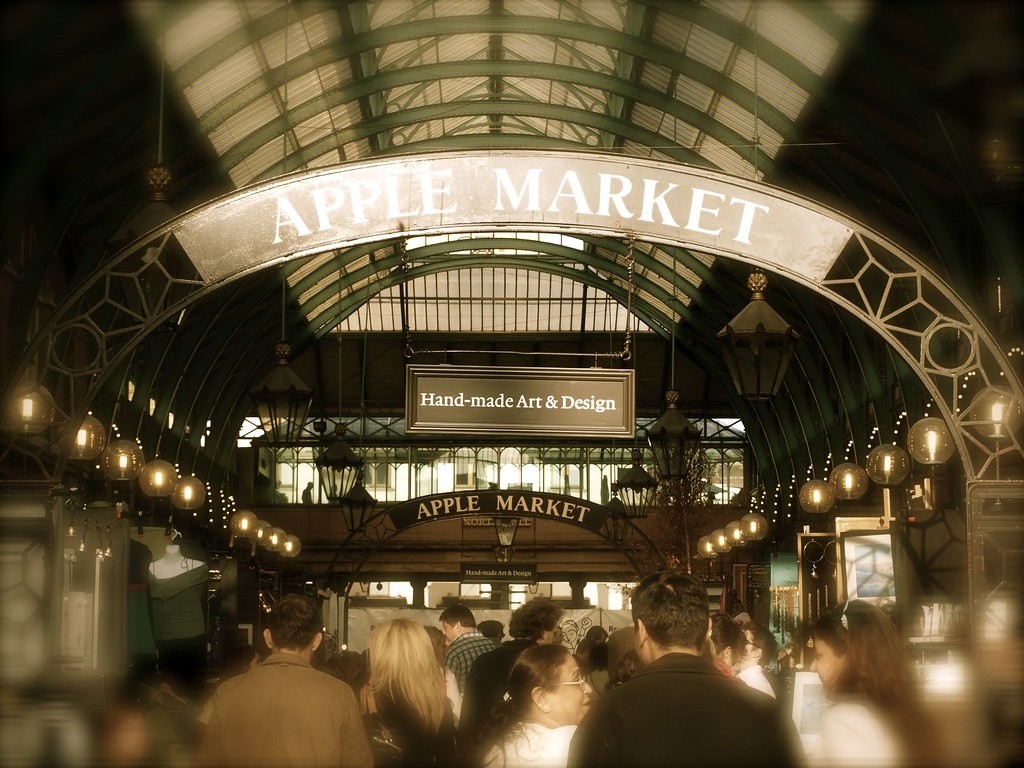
[552,671,587,686]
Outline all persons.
[111,567,1024,768]
[302,482,314,504]
[143,543,208,667]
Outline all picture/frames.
[839,529,894,622]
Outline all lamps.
[0,361,300,570]
[106,11,188,333]
[714,0,802,403]
[696,385,1024,583]
[339,254,378,533]
[645,246,702,479]
[248,0,316,448]
[313,251,363,502]
[492,515,522,565]
[618,264,659,519]
[61,479,130,565]
